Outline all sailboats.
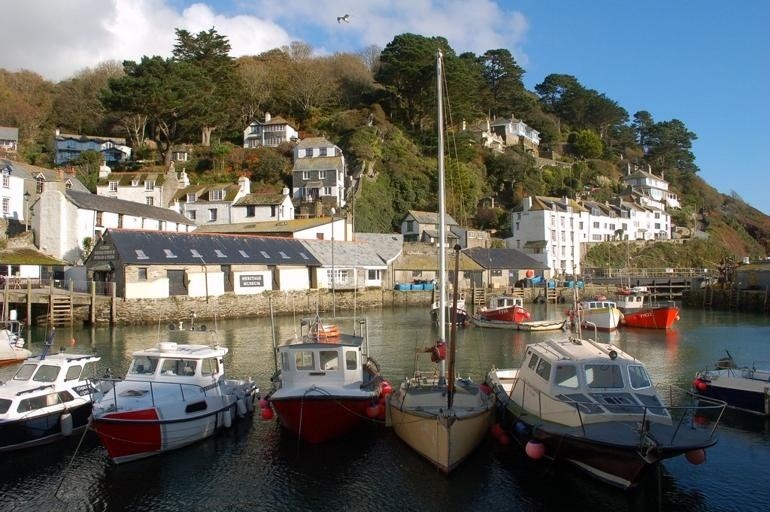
[386,46,500,477]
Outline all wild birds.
[337,14,350,24]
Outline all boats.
[577,327,620,346]
[426,270,683,333]
[0,326,123,459]
[688,364,769,440]
[477,262,729,499]
[82,314,261,470]
[254,204,394,451]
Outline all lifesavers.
[312,324,340,339]
